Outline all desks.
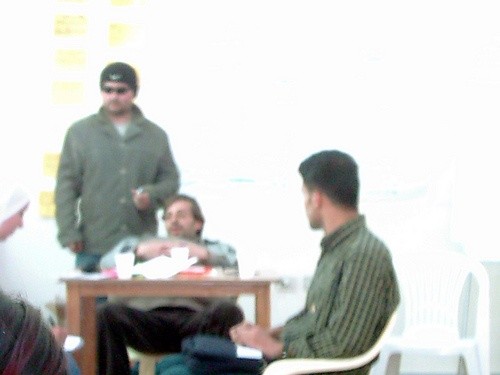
[61,278,272,375]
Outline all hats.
[100,62,137,91]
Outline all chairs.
[261,294,403,375]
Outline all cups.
[115,253,136,280]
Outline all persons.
[154,151,399,375]
[0,289,69,375]
[97,194,243,375]
[55,62,180,304]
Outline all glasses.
[102,87,129,94]
